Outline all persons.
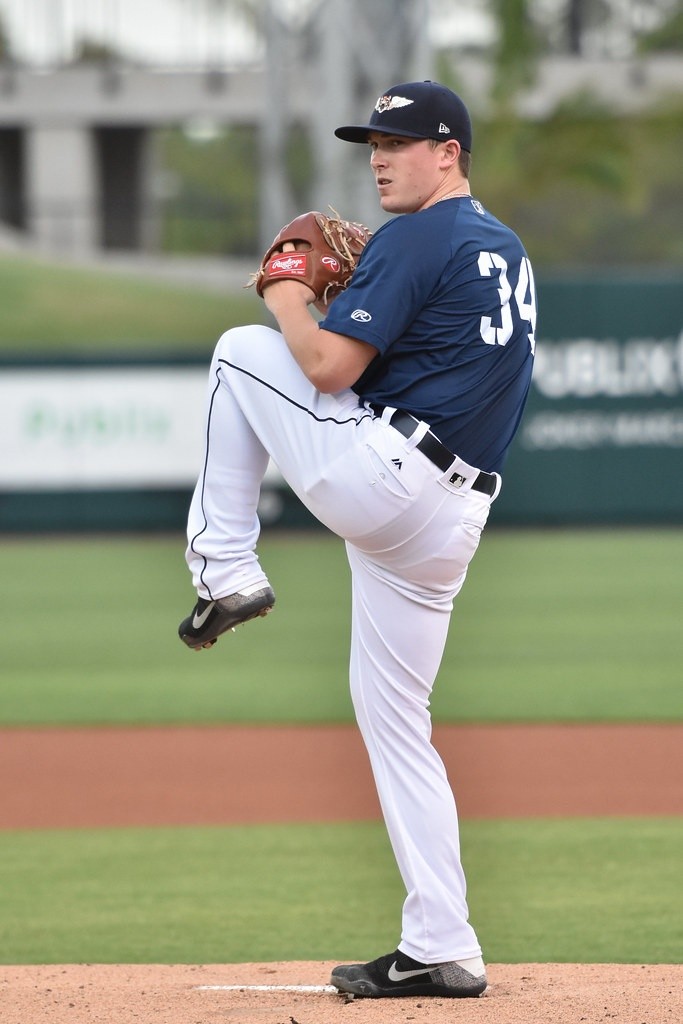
[180,78,540,1000]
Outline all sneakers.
[178,579,275,651]
[330,949,487,997]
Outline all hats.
[334,80,471,153]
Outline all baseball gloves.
[243,205,372,314]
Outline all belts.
[374,405,496,495]
